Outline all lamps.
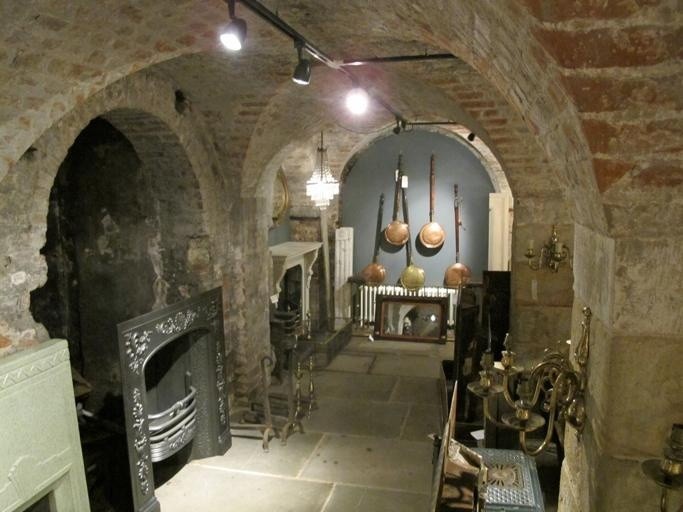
[289,34,312,87]
[305,129,340,210]
[216,2,250,52]
